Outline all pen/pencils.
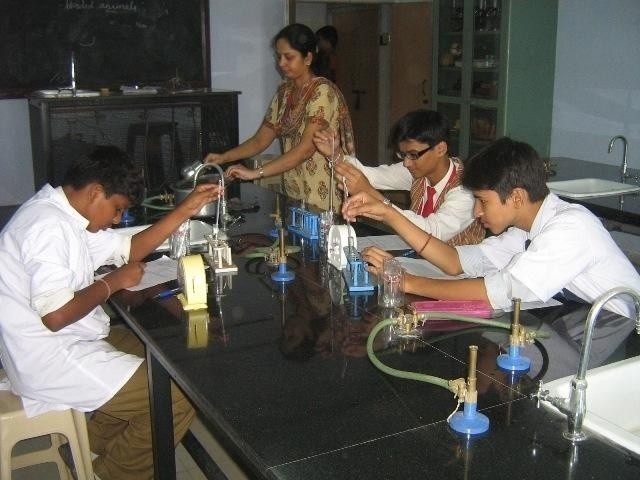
[121,255,129,265]
[152,287,180,299]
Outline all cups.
[318,213,337,251]
[168,217,191,259]
[376,256,406,308]
[376,304,403,343]
[320,250,328,280]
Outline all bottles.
[472,41,497,139]
[448,0,500,32]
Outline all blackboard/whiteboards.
[0,0,211,100]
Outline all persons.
[0,144,226,480]
[280,262,393,361]
[466,305,637,425]
[341,136,640,321]
[202,23,356,218]
[310,24,338,84]
[312,108,486,248]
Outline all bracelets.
[416,232,433,257]
[384,198,391,206]
[326,151,341,163]
[258,166,264,179]
[98,279,111,303]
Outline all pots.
[168,170,229,218]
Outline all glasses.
[396,145,435,160]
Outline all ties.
[422,186,436,217]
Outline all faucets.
[530,287,639,442]
[192,163,243,237]
[608,134,639,183]
[58,51,78,97]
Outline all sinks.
[113,220,230,251]
[545,356,639,455]
[547,178,640,198]
[40,89,102,98]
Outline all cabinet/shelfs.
[430,1,558,163]
[25,87,241,192]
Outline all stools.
[0,368,94,480]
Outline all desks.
[93,232,640,480]
